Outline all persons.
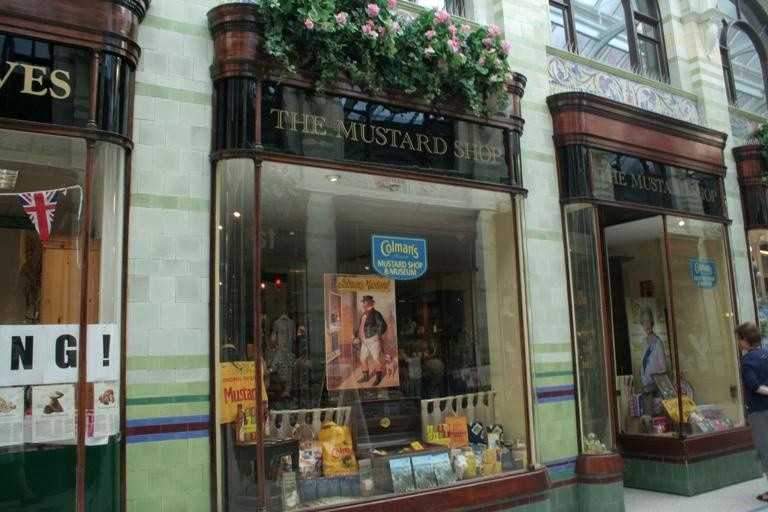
[270,306,297,400]
[735,323,768,501]
[354,296,388,386]
[639,305,667,393]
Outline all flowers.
[258,0,514,115]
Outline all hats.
[361,295,375,302]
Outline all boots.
[373,373,382,386]
[357,370,370,382]
